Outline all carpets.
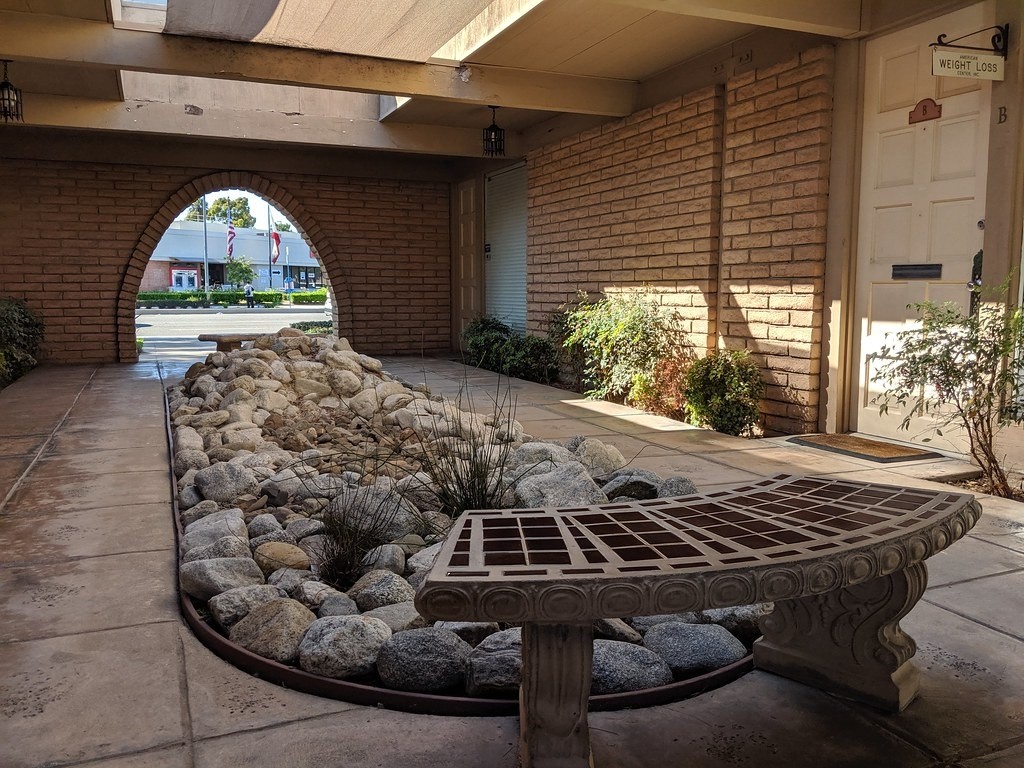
[787,433,945,464]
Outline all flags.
[227,211,236,255]
[269,212,281,264]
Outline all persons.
[242,280,255,308]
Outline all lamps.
[0,59,25,123]
[481,105,506,158]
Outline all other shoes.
[248,306,250,308]
[252,306,254,308]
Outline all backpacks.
[245,290,249,295]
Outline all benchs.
[198,333,327,352]
[413,471,984,767]
